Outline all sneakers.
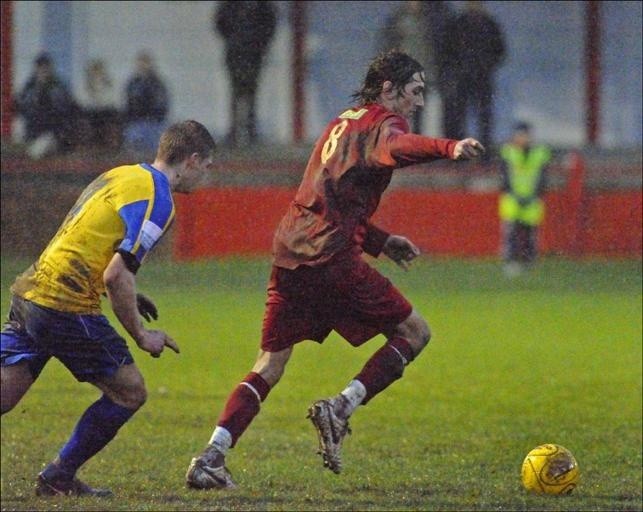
[34,471,115,498]
[186,457,236,489]
[306,400,351,474]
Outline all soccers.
[519,444,580,497]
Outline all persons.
[1,120,216,496]
[210,0,281,149]
[370,1,511,167]
[7,51,173,159]
[185,45,486,490]
[492,122,555,278]
